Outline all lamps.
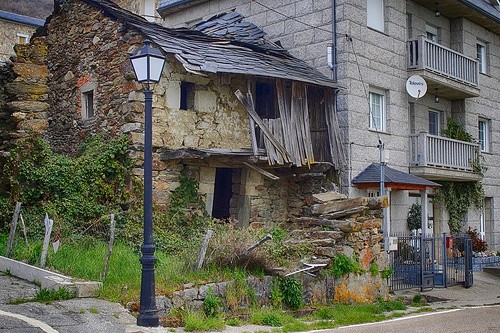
[434,87,440,104]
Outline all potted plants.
[407,202,431,233]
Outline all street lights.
[129,38,167,329]
